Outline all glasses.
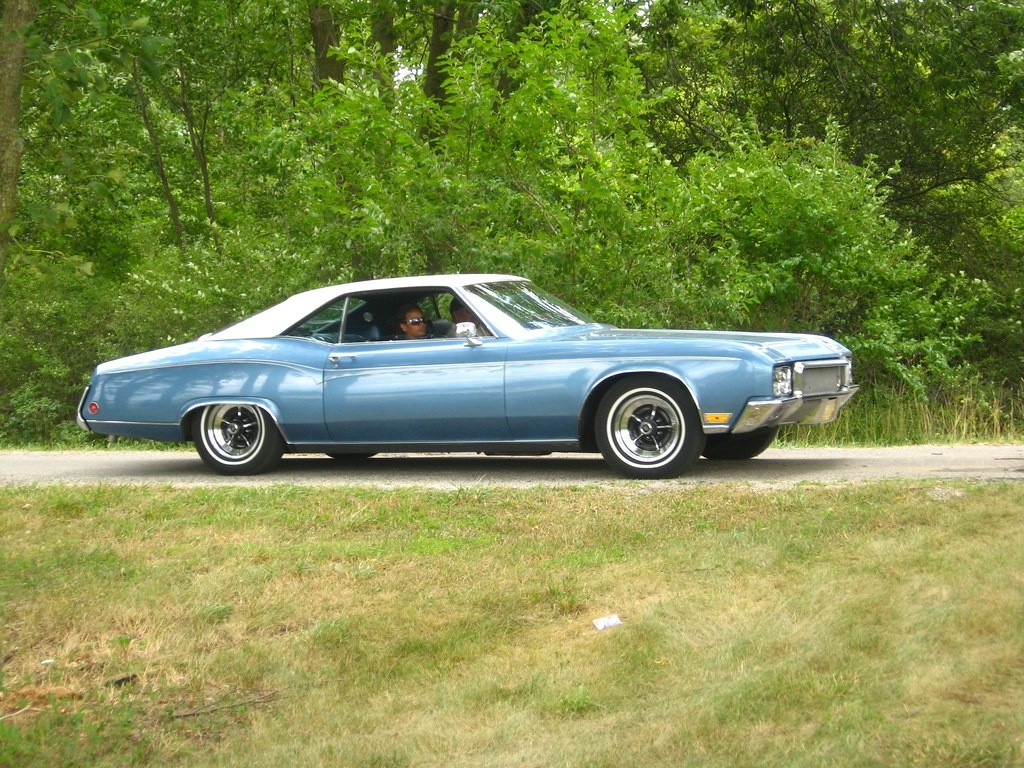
[400,317,425,325]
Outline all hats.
[450,299,464,312]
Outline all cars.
[74,270,864,481]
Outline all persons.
[445,297,491,337]
[394,302,447,339]
